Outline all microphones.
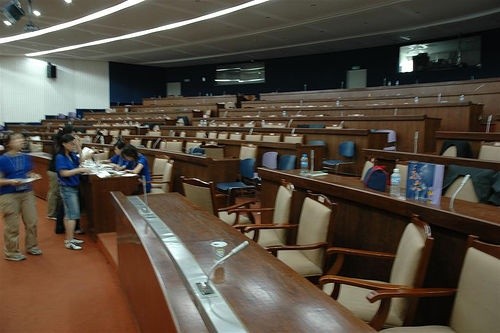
[142,176,149,214]
[204,240,250,291]
[450,175,472,209]
[311,150,315,174]
[87,77,492,149]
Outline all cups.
[211,241,228,269]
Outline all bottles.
[300,154,308,176]
[390,169,401,198]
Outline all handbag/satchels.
[364,165,388,192]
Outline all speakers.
[2,1,25,24]
[47,65,56,78]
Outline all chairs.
[27,121,500,333]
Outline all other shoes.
[74,229,82,234]
[65,238,84,244]
[65,242,82,250]
[27,246,42,255]
[48,216,56,220]
[4,253,26,261]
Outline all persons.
[47,126,152,250]
[0,133,43,261]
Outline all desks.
[7,78,500,234]
[108,190,380,333]
[257,166,500,325]
